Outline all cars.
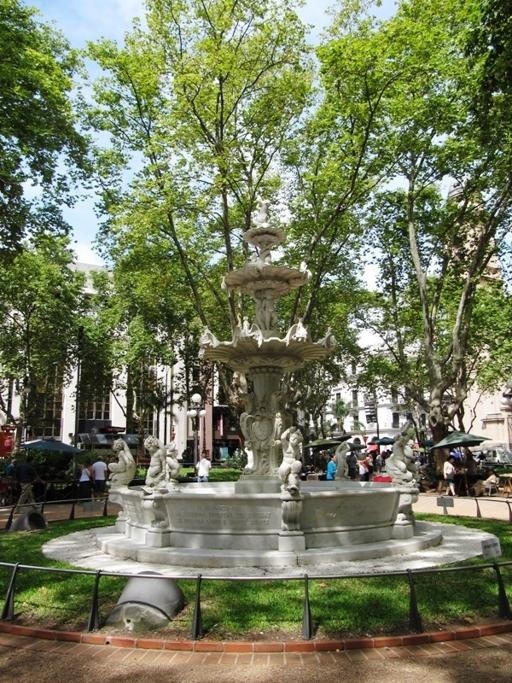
[449,446,512,487]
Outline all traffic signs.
[363,402,377,424]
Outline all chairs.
[482,478,500,496]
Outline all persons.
[195,451,212,482]
[106,437,137,488]
[79,452,118,501]
[392,429,418,481]
[143,435,168,493]
[164,441,180,482]
[302,449,500,497]
[278,426,304,496]
[14,456,47,513]
[5,459,18,478]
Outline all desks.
[499,473,512,498]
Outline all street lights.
[185,392,208,466]
[90,426,97,453]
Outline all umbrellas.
[428,430,493,495]
[303,436,394,468]
